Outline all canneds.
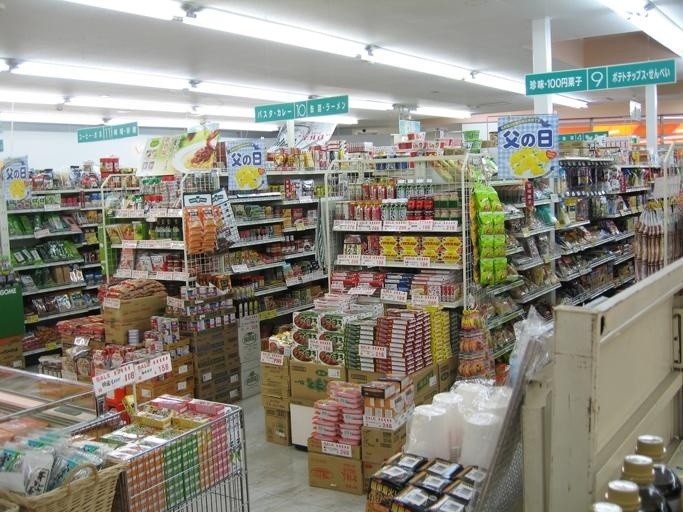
[334,182,461,227]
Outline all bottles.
[265,234,295,264]
[335,177,408,220]
[147,218,183,241]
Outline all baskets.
[0,456,126,512]
